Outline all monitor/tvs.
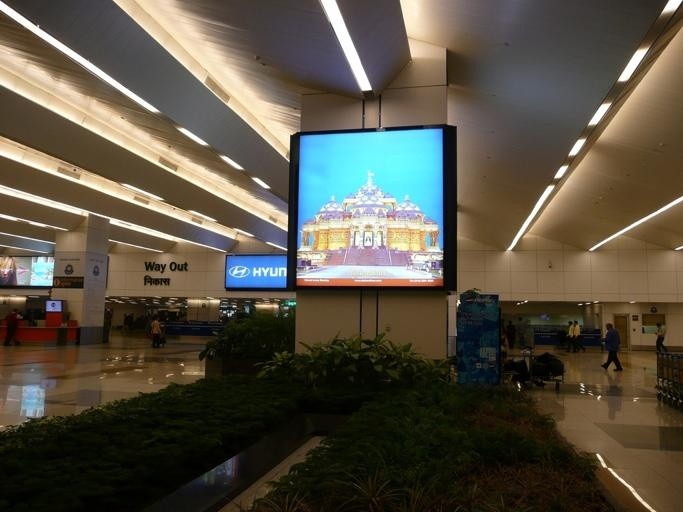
[46,300,63,312]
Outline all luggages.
[532,352,564,378]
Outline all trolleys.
[523,354,564,393]
[151,322,166,349]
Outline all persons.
[3,306,178,348]
[599,323,623,372]
[654,324,668,352]
[499,316,586,354]
